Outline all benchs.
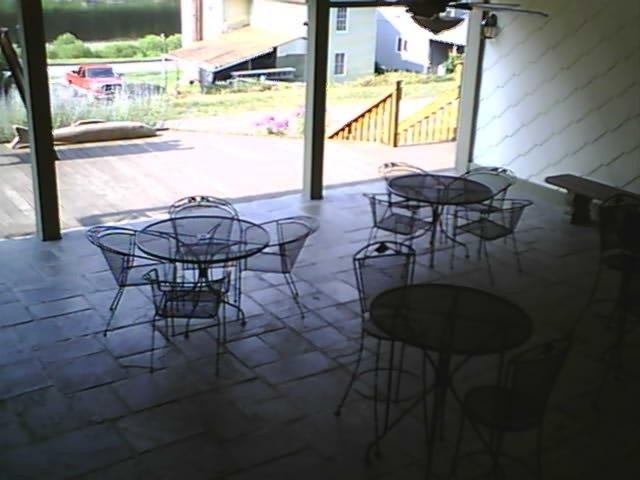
[544,171,640,227]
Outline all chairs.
[589,190,640,301]
[335,239,437,420]
[442,164,518,252]
[168,194,241,306]
[363,190,437,271]
[135,214,271,327]
[86,224,178,338]
[451,337,572,477]
[378,159,446,241]
[237,213,321,321]
[142,267,232,378]
[447,194,535,275]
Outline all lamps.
[407,11,465,35]
[479,13,500,39]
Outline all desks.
[367,282,535,475]
[386,170,496,260]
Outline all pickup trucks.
[66,64,126,105]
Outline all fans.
[328,0,551,19]
